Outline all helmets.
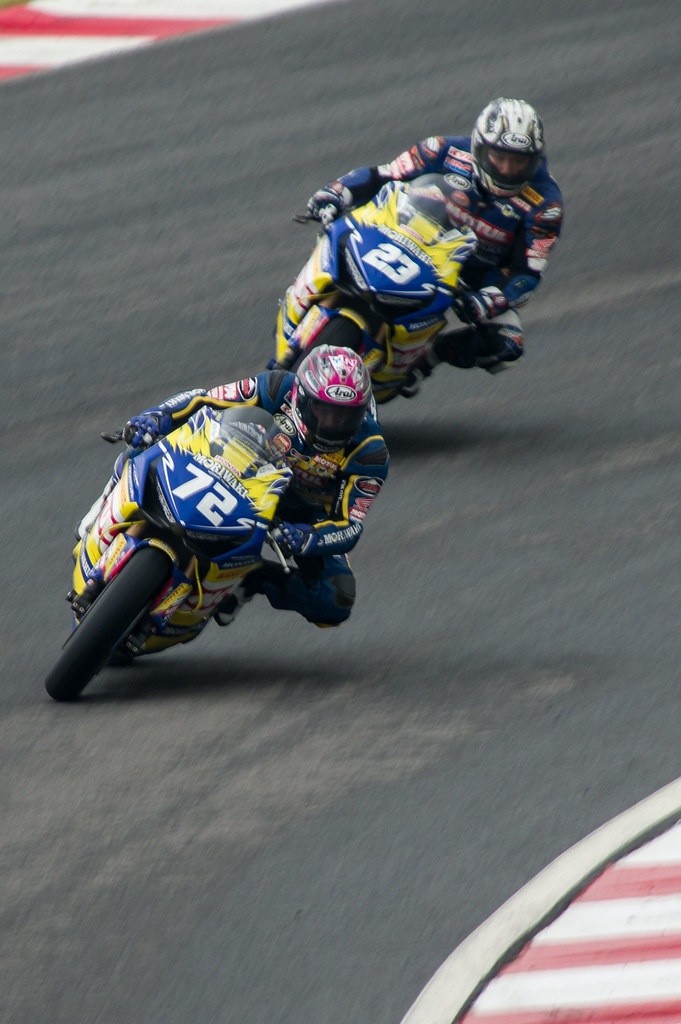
[291,343,372,454]
[471,97,545,197]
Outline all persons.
[307,97,565,398]
[77,344,390,628]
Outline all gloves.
[124,408,173,449]
[268,521,318,558]
[452,284,509,325]
[308,180,354,226]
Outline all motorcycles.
[41,401,295,706]
[266,174,471,411]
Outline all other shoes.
[76,479,112,541]
[213,574,252,627]
[405,333,443,387]
